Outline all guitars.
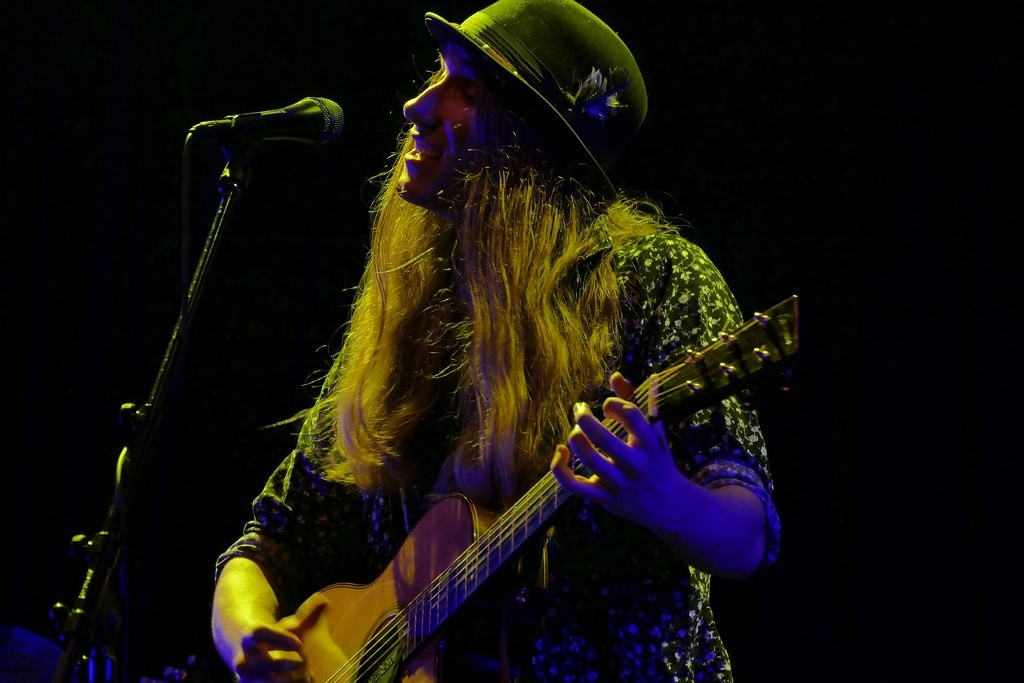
[293,294,808,683]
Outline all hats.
[423,1,648,208]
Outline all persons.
[208,0,783,682]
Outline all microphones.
[189,97,346,146]
